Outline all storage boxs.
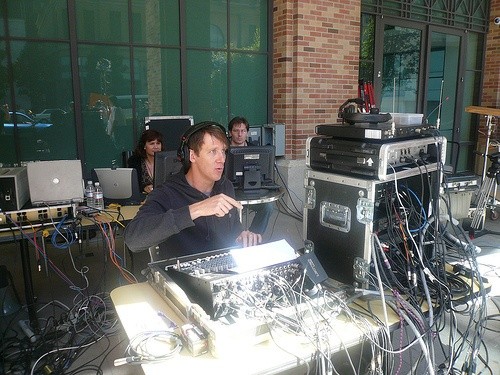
[379,112,423,126]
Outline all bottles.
[93,182,104,210]
[86,181,94,207]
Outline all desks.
[110,263,491,375]
[0,186,285,330]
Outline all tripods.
[469,161,500,240]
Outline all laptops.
[91,168,141,206]
[26,159,84,207]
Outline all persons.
[105,96,124,151]
[128,128,163,194]
[228,118,256,146]
[124,121,263,262]
[0,104,10,136]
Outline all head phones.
[341,105,392,125]
[177,121,228,165]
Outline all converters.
[296,252,328,289]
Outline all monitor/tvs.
[153,151,183,190]
[225,145,275,195]
